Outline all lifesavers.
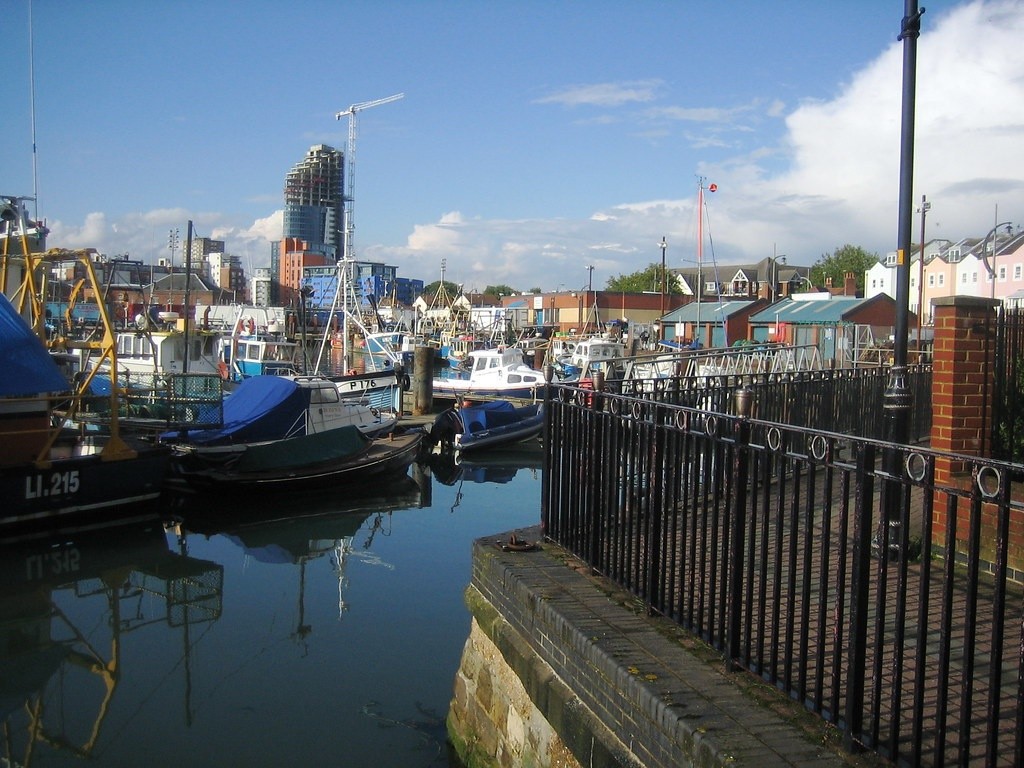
[399,374,411,391]
[238,315,255,337]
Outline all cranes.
[335,92,407,310]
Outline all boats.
[0,195,717,553]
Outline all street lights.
[914,195,930,367]
[585,265,596,290]
[656,236,668,317]
